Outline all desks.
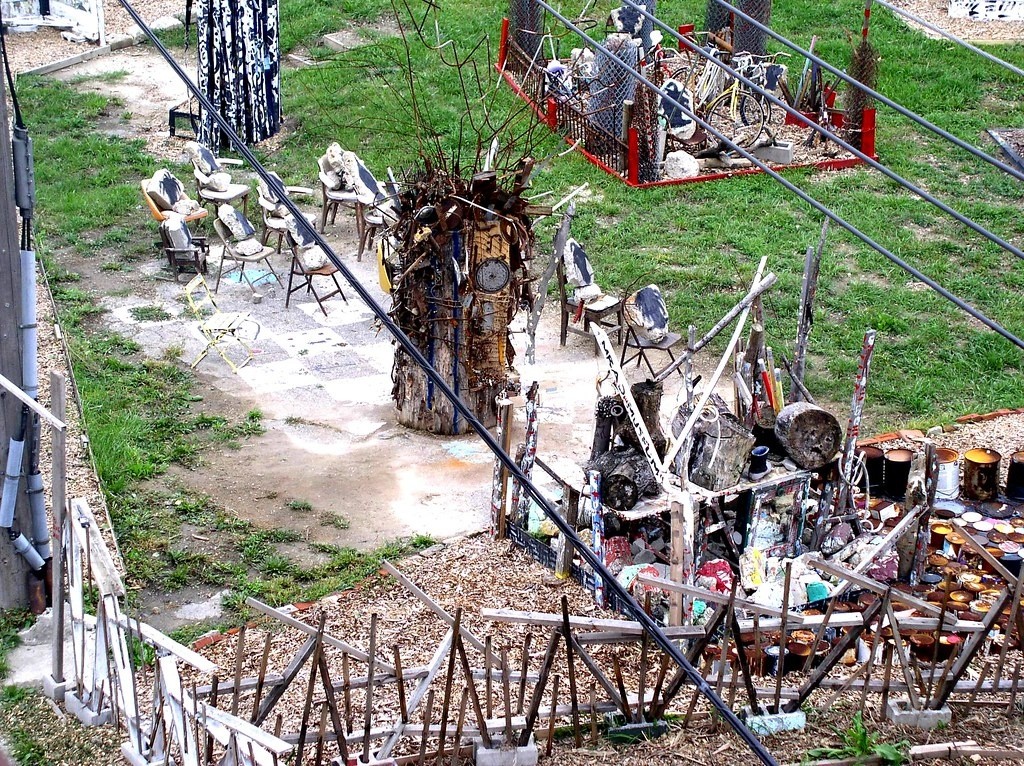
[604,457,816,572]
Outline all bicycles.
[665,27,791,151]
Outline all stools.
[356,207,386,263]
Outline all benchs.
[168,93,200,136]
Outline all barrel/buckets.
[702,445,1024,678]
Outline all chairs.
[183,272,262,376]
[256,186,317,256]
[212,218,285,297]
[554,242,626,358]
[191,158,249,232]
[159,219,210,283]
[283,228,348,319]
[316,155,368,239]
[618,278,685,381]
[140,177,208,249]
